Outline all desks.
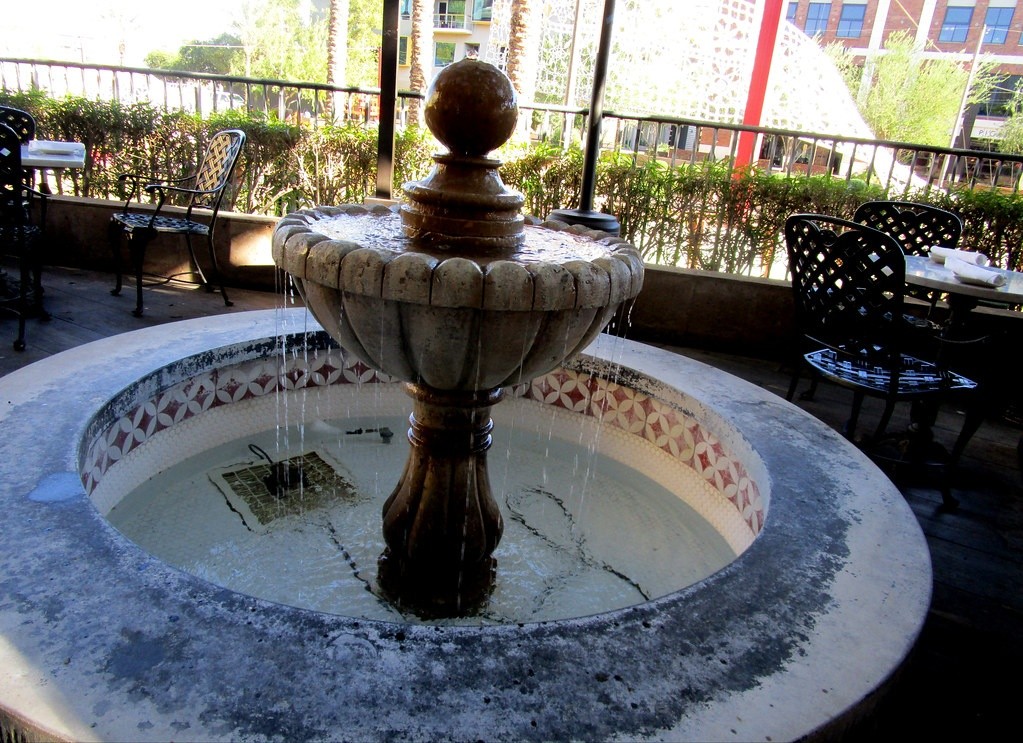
[0,140,87,323]
[866,255,1023,517]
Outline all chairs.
[846,201,946,456]
[786,213,979,509]
[0,124,36,352]
[109,129,246,316]
[0,106,40,288]
